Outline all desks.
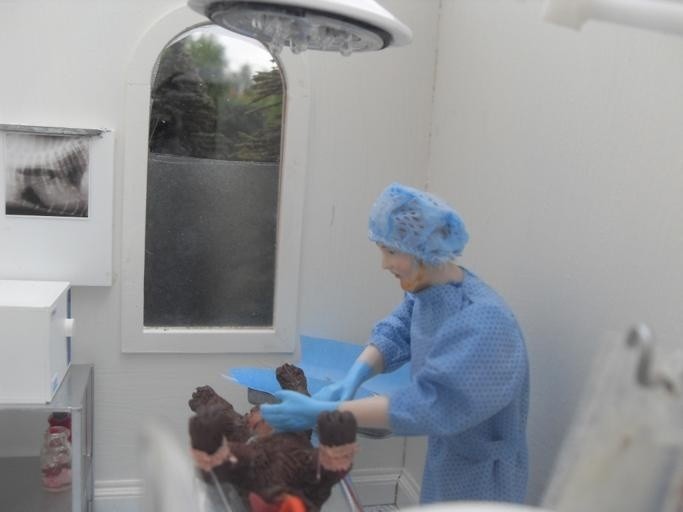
[0,364,95,511]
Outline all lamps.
[187,0,413,60]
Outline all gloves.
[311,360,377,401]
[259,390,337,433]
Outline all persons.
[258,181,534,512]
